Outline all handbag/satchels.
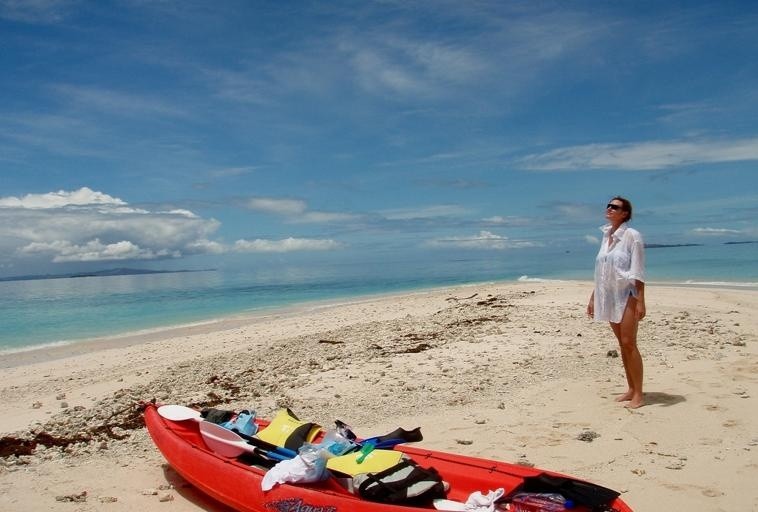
[224,412,257,436]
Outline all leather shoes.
[379,427,423,442]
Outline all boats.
[143,402,637,512]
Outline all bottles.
[510,492,573,511]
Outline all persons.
[585,196,647,409]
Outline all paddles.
[157,405,299,459]
[200,421,290,462]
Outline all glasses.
[607,204,621,210]
